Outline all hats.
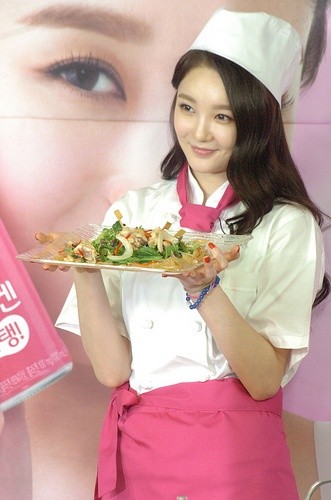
[188,8,301,108]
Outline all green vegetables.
[92,220,200,264]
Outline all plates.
[16,222,253,274]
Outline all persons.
[0,0,330,500]
[34,25,327,500]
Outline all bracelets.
[185,275,221,312]
